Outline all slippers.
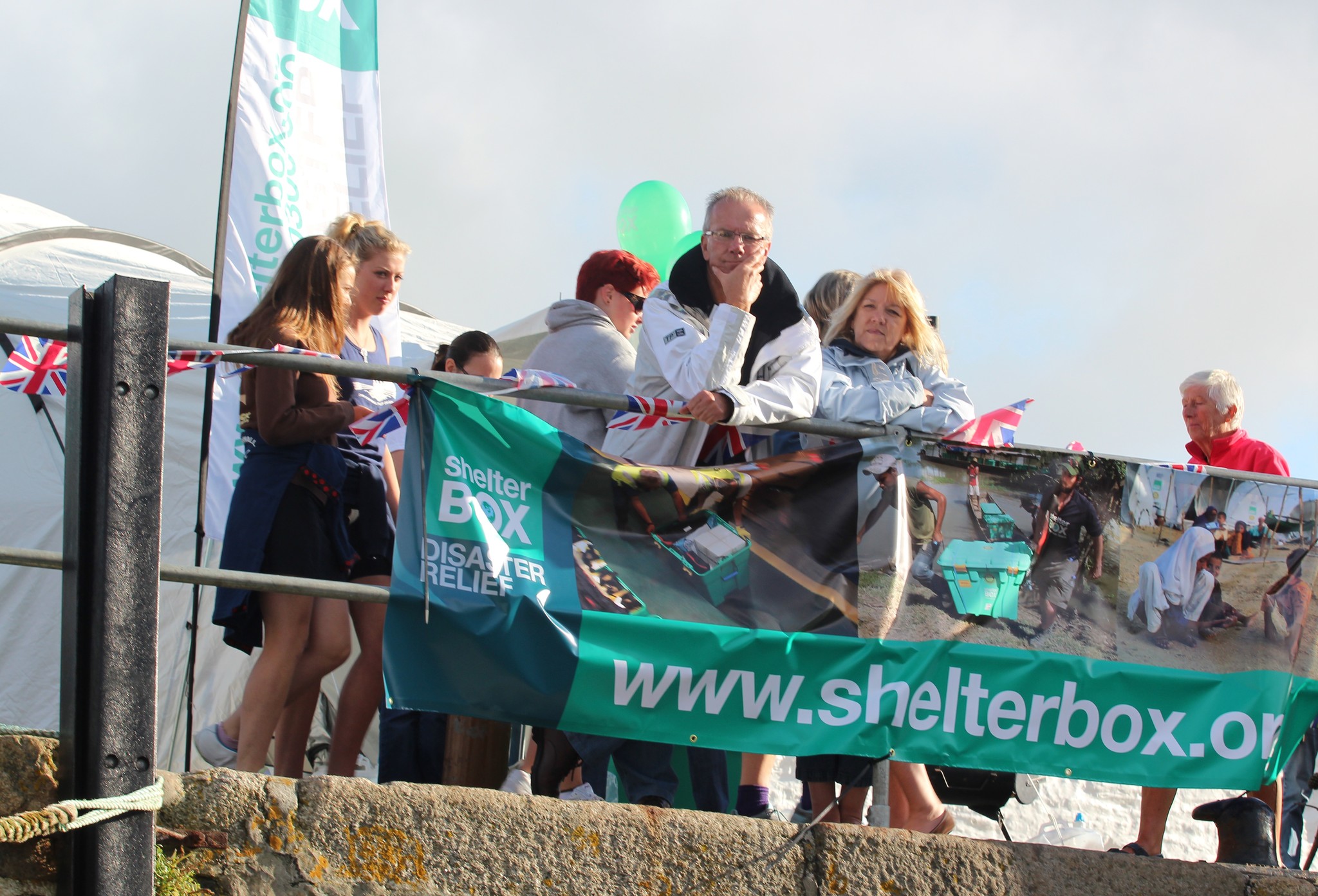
[1106,842,1164,857]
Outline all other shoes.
[1169,628,1197,648]
[192,726,312,778]
[1153,632,1173,650]
[790,796,813,823]
[499,766,533,794]
[558,782,605,802]
[929,809,955,835]
[731,804,789,823]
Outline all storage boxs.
[651,509,752,606]
[979,503,1003,523]
[936,538,1034,621]
[983,514,1015,538]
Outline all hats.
[1057,454,1085,477]
[862,453,897,476]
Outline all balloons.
[617,181,703,280]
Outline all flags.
[0,335,68,396]
[167,349,224,375]
[350,389,412,447]
[607,394,693,431]
[726,424,779,456]
[199,0,404,542]
[943,398,1033,449]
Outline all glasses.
[616,287,647,315]
[706,228,768,246]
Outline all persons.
[612,464,688,533]
[500,250,661,798]
[773,269,864,455]
[799,268,976,835]
[857,453,954,609]
[967,457,980,510]
[194,216,410,774]
[1128,527,1259,649]
[1262,547,1311,666]
[601,187,823,821]
[686,469,752,540]
[432,330,503,379]
[1193,506,1271,558]
[1028,454,1103,646]
[212,235,356,778]
[1106,369,1288,871]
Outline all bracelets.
[647,522,654,526]
